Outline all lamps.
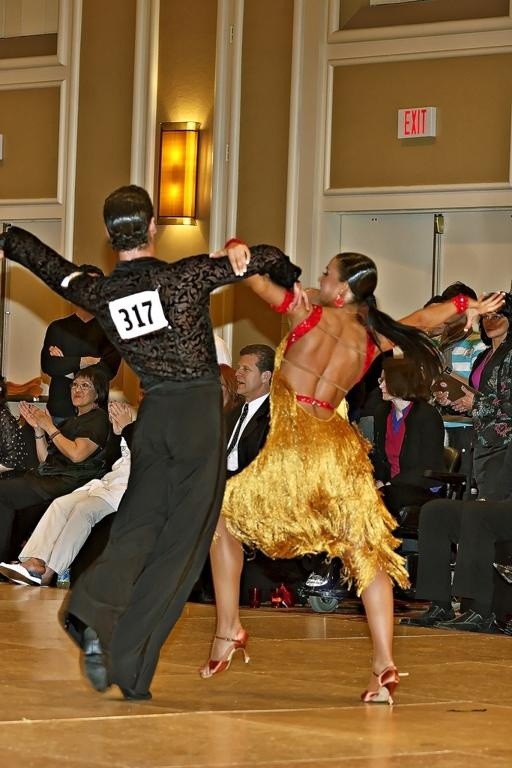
[154,116,206,232]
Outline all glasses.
[70,381,93,390]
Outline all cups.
[248,586,261,609]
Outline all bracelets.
[224,238,246,250]
[451,291,469,314]
[35,435,45,439]
[50,431,61,440]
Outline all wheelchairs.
[298,445,467,613]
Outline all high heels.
[361,666,401,705]
[200,630,249,678]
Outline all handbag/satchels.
[430,368,483,413]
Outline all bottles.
[271,587,283,609]
[56,566,71,589]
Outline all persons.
[1,185,311,702]
[2,376,31,479]
[219,344,276,473]
[306,282,510,633]
[0,400,136,586]
[198,236,508,705]
[41,265,122,444]
[2,367,112,562]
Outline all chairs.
[0,392,512,617]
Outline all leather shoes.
[81,626,153,698]
[400,606,455,626]
[433,609,497,633]
[0,562,42,585]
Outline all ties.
[227,404,248,455]
